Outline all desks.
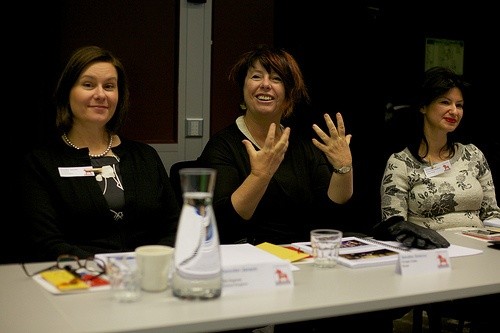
[0,231,500,333]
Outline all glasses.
[21,255,106,282]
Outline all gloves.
[390,221,451,249]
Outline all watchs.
[333,165,353,174]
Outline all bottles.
[171,168,223,301]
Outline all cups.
[107,255,145,303]
[310,228,342,268]
[135,245,173,292]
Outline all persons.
[21,45,183,263]
[381,66,500,333]
[198,47,393,332]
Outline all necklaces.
[63,132,113,157]
